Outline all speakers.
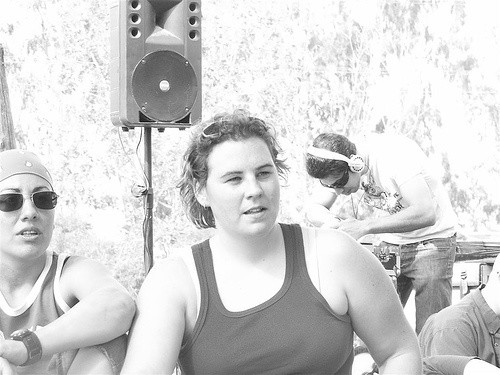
[110,0,202,132]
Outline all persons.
[120,105,422,375]
[418,250,500,375]
[0,148,136,375]
[300,130,455,375]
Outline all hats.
[0,148,58,192]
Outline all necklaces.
[351,191,365,220]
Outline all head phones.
[306,146,366,174]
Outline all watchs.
[10,327,43,366]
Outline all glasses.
[320,168,349,189]
[190,116,268,157]
[0,191,60,213]
